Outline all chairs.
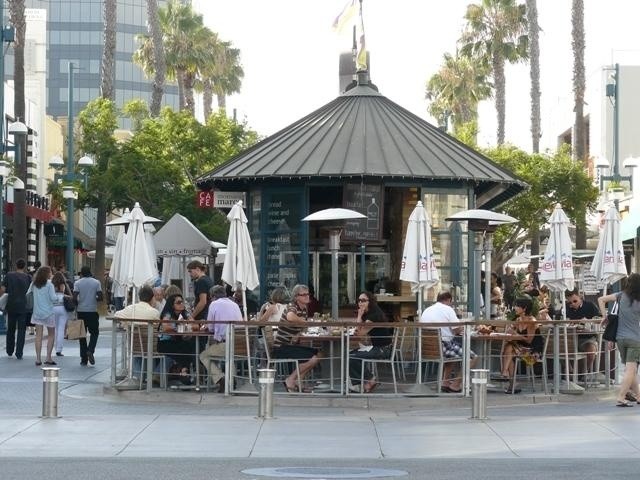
[107,290,619,401]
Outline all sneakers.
[56,352,64,356]
[142,365,383,394]
[566,371,594,383]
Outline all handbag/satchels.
[25,291,33,310]
[63,294,75,311]
[64,319,87,340]
[602,292,622,342]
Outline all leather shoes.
[79,350,95,366]
[7,351,23,359]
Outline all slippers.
[492,373,509,382]
[505,387,521,394]
[441,382,461,393]
[34,358,57,366]
[615,390,640,407]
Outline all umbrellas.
[162,255,190,298]
[113,202,160,390]
[220,199,261,395]
[110,208,132,380]
[582,198,627,384]
[399,200,440,396]
[540,202,586,394]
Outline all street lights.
[595,62,637,292]
[1,0,31,334]
[49,62,95,285]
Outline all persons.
[480,270,640,406]
[114,260,323,393]
[349,291,393,393]
[0,259,103,365]
[420,291,477,393]
[111,280,129,329]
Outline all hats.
[78,266,93,276]
